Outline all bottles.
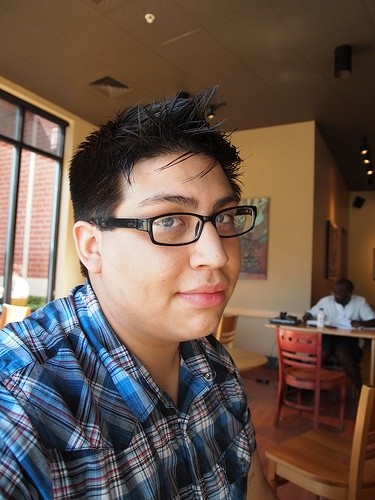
[317,308,325,329]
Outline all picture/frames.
[327,220,338,281]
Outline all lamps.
[334,45,351,80]
[206,106,215,119]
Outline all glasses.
[93,202,258,246]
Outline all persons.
[303,276,375,398]
[0,82,257,499]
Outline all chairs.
[265,384,375,499]
[0,303,31,330]
[216,314,238,352]
[273,323,364,431]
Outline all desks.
[270,320,375,388]
